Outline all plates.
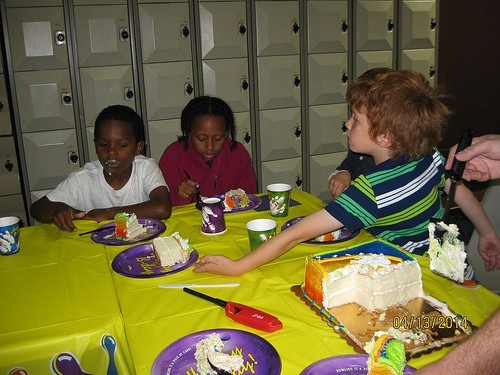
[298,353,418,375]
[281,216,360,244]
[112,242,199,278]
[196,194,263,214]
[150,329,282,375]
[91,217,166,245]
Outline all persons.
[191,67,500,278]
[30,104,171,231]
[158,96,257,206]
[414,136,500,374]
[329,151,376,198]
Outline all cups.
[245,219,277,251]
[0,216,21,256]
[266,183,293,217]
[199,198,227,236]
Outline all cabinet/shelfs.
[0,0,439,230]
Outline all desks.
[0,190,500,375]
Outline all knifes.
[444,128,473,215]
[160,284,239,288]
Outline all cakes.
[223,188,249,211]
[315,230,340,241]
[194,332,244,375]
[366,335,406,375]
[114,212,148,241]
[428,217,467,282]
[304,238,425,313]
[152,232,190,267]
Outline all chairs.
[465,185,500,295]
